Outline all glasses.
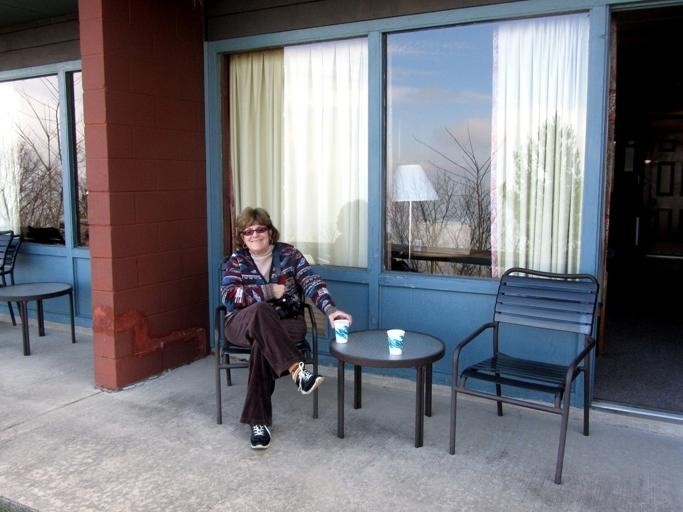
[243,227,267,235]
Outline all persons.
[328,198,415,271]
[218,204,354,451]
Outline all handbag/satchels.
[267,294,298,319]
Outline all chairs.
[448,264,602,488]
[412,219,473,276]
[0,229,26,326]
[214,255,319,424]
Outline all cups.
[334,320,349,343]
[386,329,405,356]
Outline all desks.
[329,330,446,448]
[392,242,493,273]
[0,281,76,356]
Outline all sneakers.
[294,367,324,395]
[249,423,272,449]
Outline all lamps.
[392,163,441,270]
